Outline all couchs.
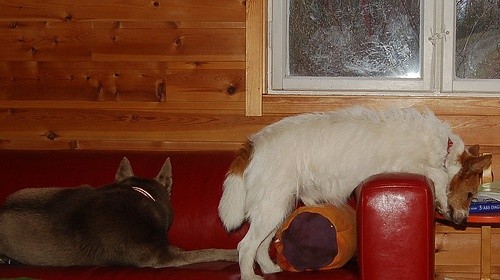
[0,150,435,280]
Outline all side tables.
[435,209,500,280]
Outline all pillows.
[268,203,358,274]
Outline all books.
[469,192,500,213]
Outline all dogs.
[1,157,239,270]
[218,104,494,280]
